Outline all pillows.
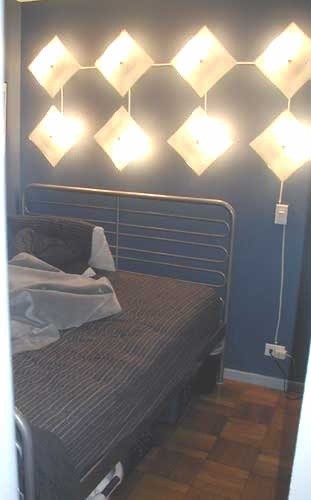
[7,213,117,273]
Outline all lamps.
[27,104,82,169]
[92,104,152,173]
[24,33,82,101]
[169,24,237,99]
[256,22,311,99]
[249,109,311,183]
[166,105,236,179]
[94,27,156,98]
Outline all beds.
[7,180,239,500]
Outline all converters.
[274,349,288,360]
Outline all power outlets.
[275,204,289,225]
[263,343,288,360]
